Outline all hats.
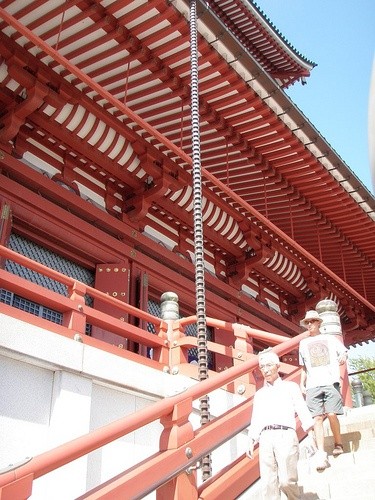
[300,310,324,328]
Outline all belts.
[260,424,288,431]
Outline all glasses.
[304,319,319,325]
[258,363,273,369]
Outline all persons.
[298,310,348,470]
[246,347,318,499]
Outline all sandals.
[315,459,331,470]
[333,442,344,456]
